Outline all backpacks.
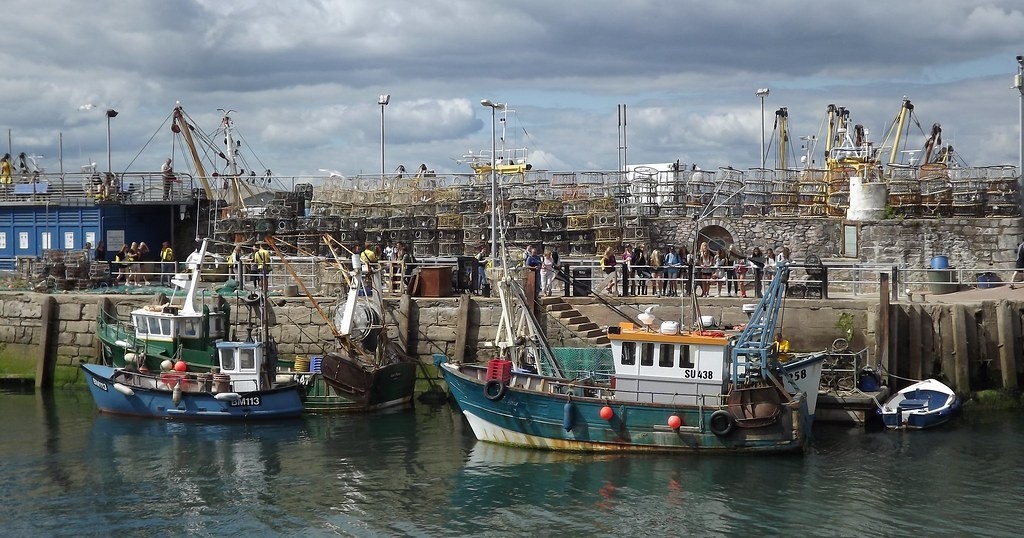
[162,246,173,262]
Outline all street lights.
[480,99,505,266]
[755,87,770,168]
[105,108,119,173]
[1009,55,1024,193]
[377,94,391,188]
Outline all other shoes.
[145,281,151,286]
[125,282,130,286]
[728,292,737,298]
[134,283,142,287]
[544,290,551,298]
[714,293,722,298]
[702,295,710,298]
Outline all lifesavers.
[483,379,506,402]
[695,329,725,337]
[708,409,733,435]
[244,290,260,306]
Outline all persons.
[162,158,172,201]
[694,242,796,297]
[0,158,13,201]
[477,247,488,289]
[774,333,789,353]
[84,242,91,258]
[94,241,106,261]
[249,244,272,289]
[1009,241,1024,288]
[523,245,555,296]
[360,239,413,290]
[232,245,242,281]
[622,244,694,298]
[116,242,151,287]
[89,175,120,194]
[160,242,174,287]
[602,247,620,294]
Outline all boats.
[94,235,420,414]
[881,378,963,429]
[432,251,827,458]
[79,341,307,424]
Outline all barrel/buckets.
[861,369,879,392]
[926,256,957,295]
[294,355,322,373]
[197,373,230,393]
